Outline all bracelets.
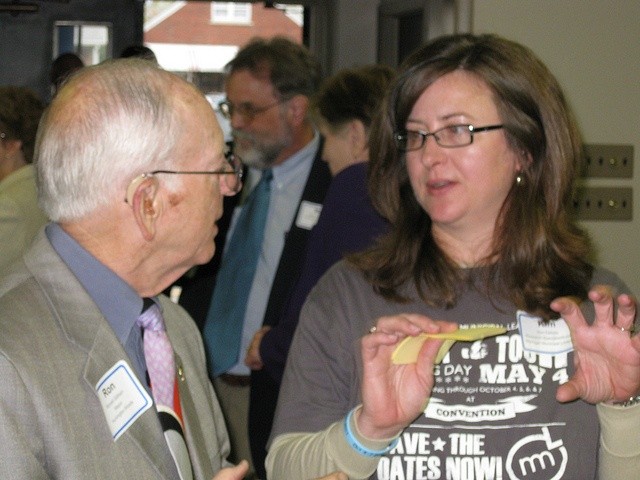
[609,393,640,408]
[343,404,401,458]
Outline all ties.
[137,300,194,480]
[205,164,272,379]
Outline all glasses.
[392,123,504,151]
[124,153,245,204]
[219,102,287,118]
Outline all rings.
[368,322,377,334]
[614,322,634,334]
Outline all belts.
[219,372,251,388]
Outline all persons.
[163,35,332,480]
[264,32,640,480]
[49,50,84,101]
[118,44,157,66]
[1,83,46,284]
[243,65,402,480]
[0,55,250,480]
